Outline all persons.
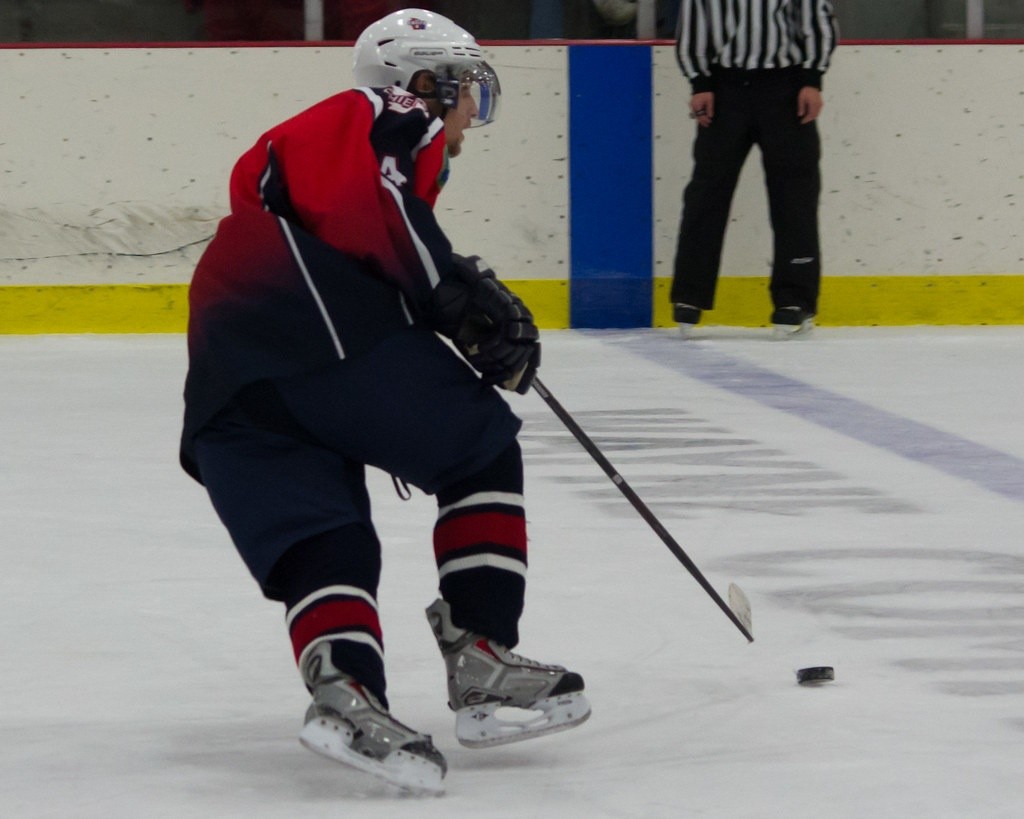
[179,6,591,799]
[666,0,839,341]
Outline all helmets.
[351,9,502,127]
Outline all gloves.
[445,270,540,395]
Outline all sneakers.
[293,638,446,798]
[423,600,591,747]
[671,300,816,333]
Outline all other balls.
[796,665,835,684]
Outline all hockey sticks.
[476,310,755,648]
